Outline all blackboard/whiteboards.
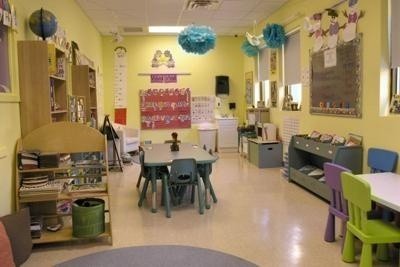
[309,33,363,118]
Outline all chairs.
[323,147,400,266]
[108,122,141,156]
[136,139,219,217]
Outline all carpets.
[51,244,258,266]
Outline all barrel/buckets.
[72,197,104,237]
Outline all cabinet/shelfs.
[287,133,364,201]
[14,39,113,246]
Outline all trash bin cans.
[198,128,217,153]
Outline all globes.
[29,7,57,41]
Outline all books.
[298,130,363,183]
[20,177,75,200]
